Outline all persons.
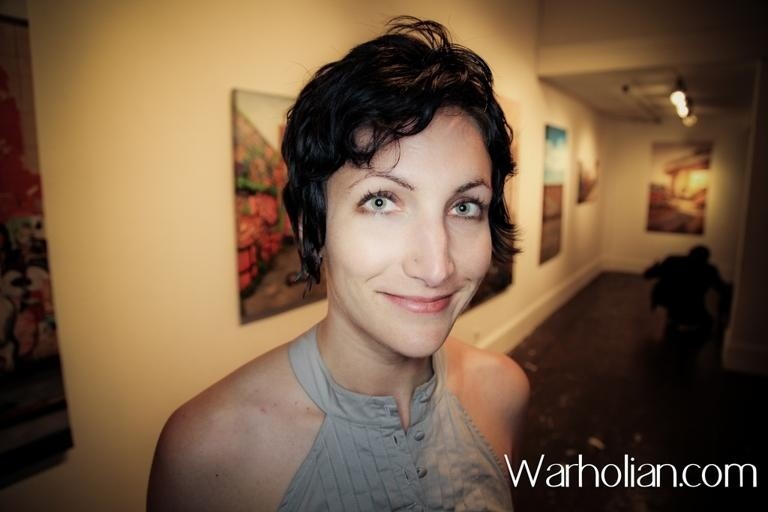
[641,244,734,361]
[145,15,533,512]
